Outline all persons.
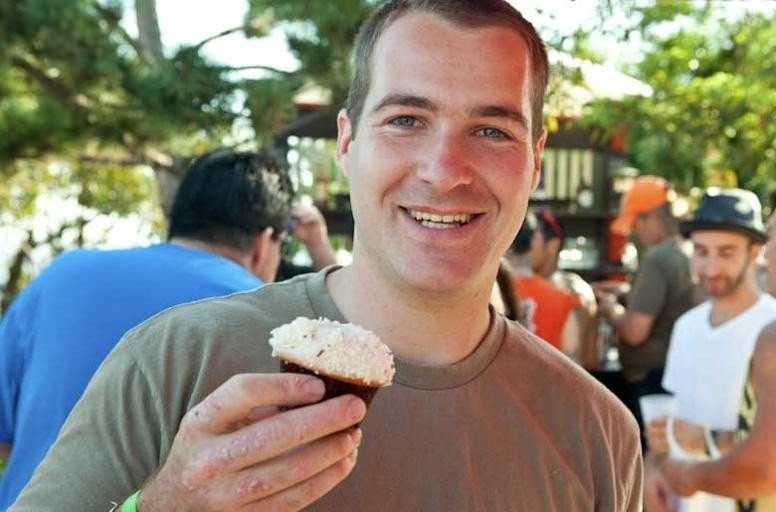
[270,184,334,282]
[0,147,289,512]
[0,1,646,511]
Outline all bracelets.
[116,489,143,512]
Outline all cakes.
[266,317,396,434]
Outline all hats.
[610,175,677,237]
[676,186,772,245]
